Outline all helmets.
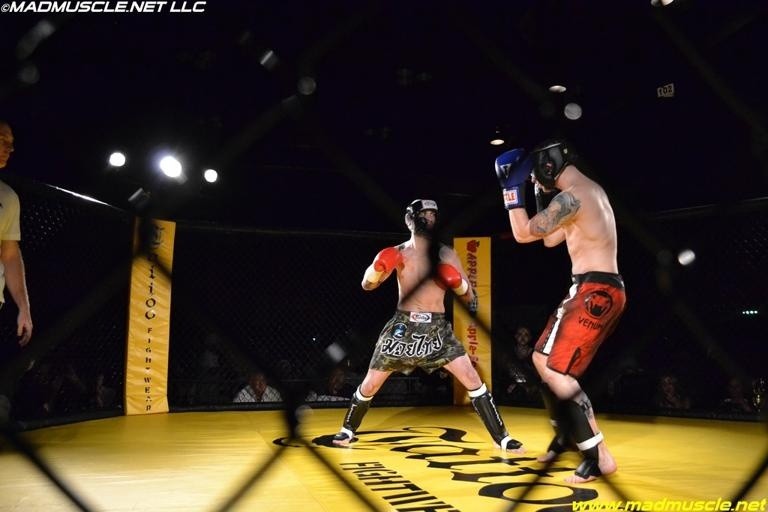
[534,140,570,190]
[406,198,439,216]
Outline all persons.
[498,317,767,419]
[0,121,34,348]
[2,346,124,422]
[494,135,626,485]
[332,197,525,452]
[162,341,454,408]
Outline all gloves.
[431,264,461,291]
[494,148,535,209]
[373,247,406,274]
